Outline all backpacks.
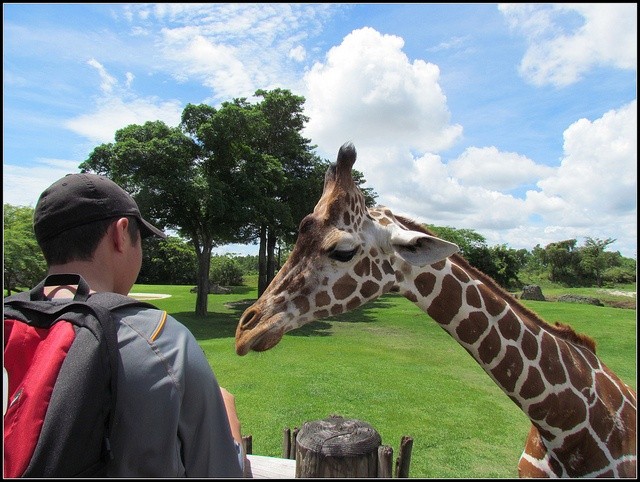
[4,273,160,479]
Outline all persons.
[33,175,252,478]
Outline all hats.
[34,173,166,242]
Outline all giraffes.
[235,142,638,479]
